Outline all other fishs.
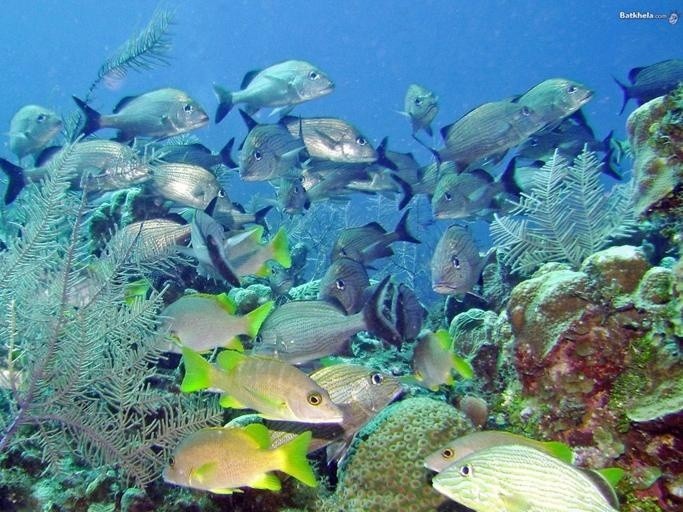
[212,60,336,125]
